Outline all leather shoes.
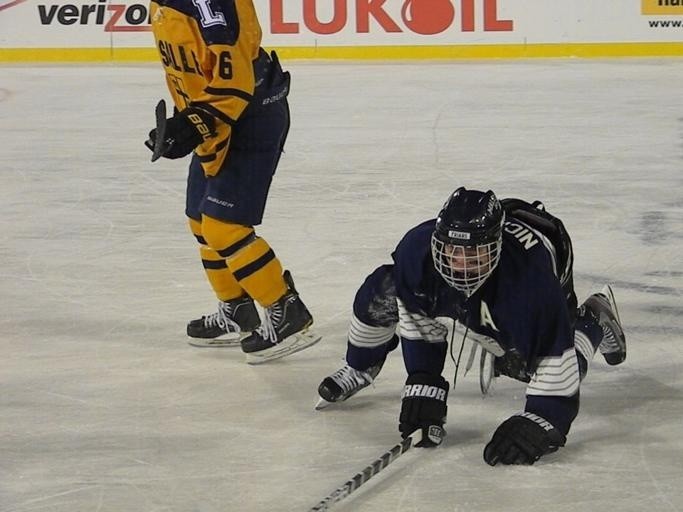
[483,411,554,466]
[398,375,448,448]
[144,107,214,159]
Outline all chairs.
[318,365,382,402]
[187,291,260,338]
[243,270,316,353]
[581,294,626,364]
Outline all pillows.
[432,186,505,292]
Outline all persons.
[317,184,626,466]
[145,0,313,354]
